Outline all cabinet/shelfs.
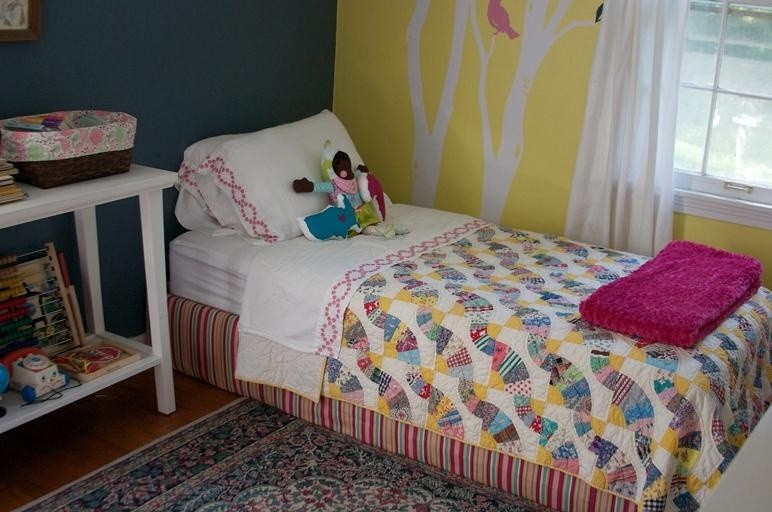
[0,165,178,434]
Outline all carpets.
[13,397,533,512]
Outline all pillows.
[175,109,366,245]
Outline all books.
[0,155,29,207]
[3,119,47,131]
[16,116,71,129]
[37,113,76,128]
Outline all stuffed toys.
[289,136,415,241]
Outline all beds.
[147,205,772,512]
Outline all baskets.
[7,148,133,190]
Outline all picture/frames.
[0,0,43,42]
